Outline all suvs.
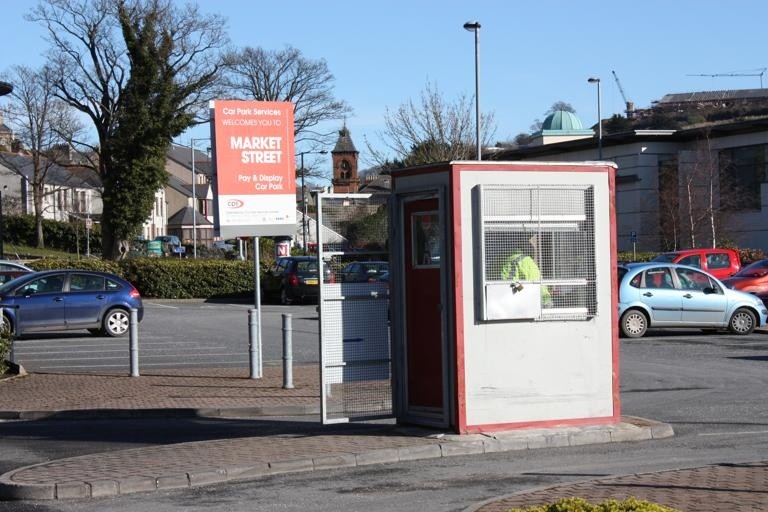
[260,255,335,306]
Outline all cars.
[339,260,389,285]
[618,259,768,338]
[0,267,144,338]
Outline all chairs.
[46,279,62,290]
[695,255,720,268]
[645,274,672,288]
[85,280,97,289]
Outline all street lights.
[463,20,482,161]
[588,78,602,160]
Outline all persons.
[497,241,554,309]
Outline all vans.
[630,248,742,289]
